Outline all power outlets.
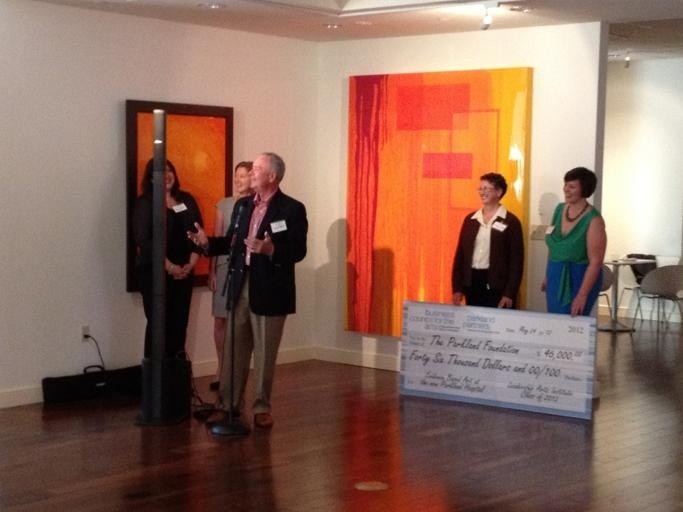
[80,323,90,342]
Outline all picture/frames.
[122,98,234,294]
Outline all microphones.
[235,204,245,228]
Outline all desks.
[596,258,654,333]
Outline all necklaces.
[566,202,588,222]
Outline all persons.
[134,158,203,363]
[187,151,306,428]
[538,167,607,315]
[209,160,256,390]
[451,172,523,312]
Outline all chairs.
[595,265,612,316]
[619,254,657,319]
[629,265,682,337]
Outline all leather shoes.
[206,410,239,427]
[255,413,274,427]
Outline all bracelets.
[168,264,174,274]
